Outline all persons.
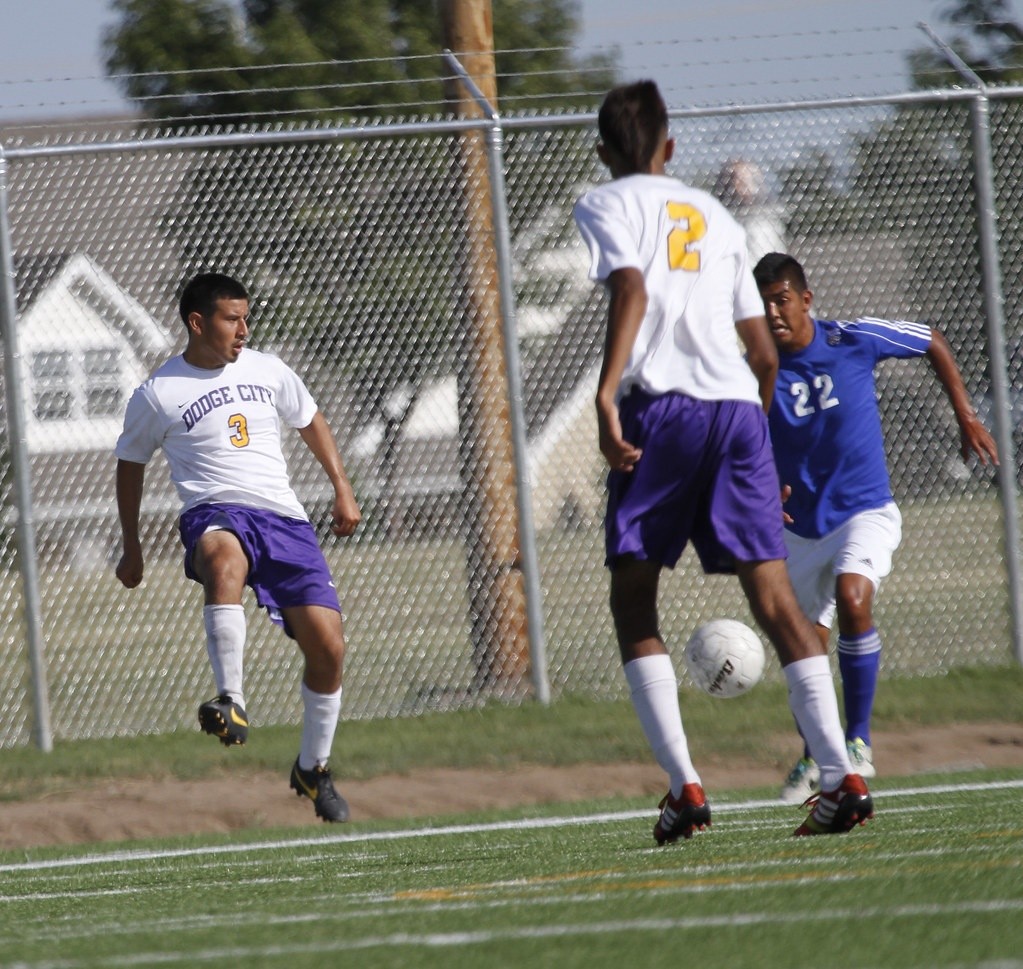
[572,79,873,845]
[750,251,1000,804]
[115,272,361,824]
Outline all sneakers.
[844,735,875,768]
[792,775,875,836]
[780,755,822,803]
[651,783,713,846]
[289,755,351,823]
[198,693,250,747]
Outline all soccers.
[685,619,765,700]
